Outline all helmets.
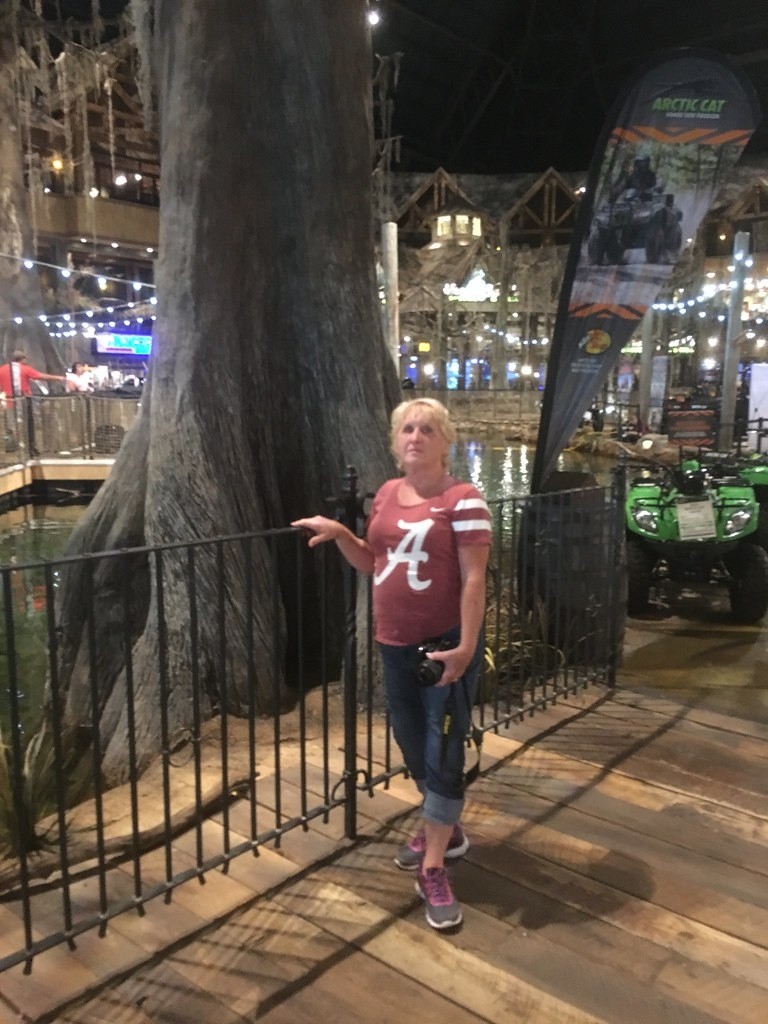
[633,155,650,168]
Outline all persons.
[291,398,494,930]
[0,350,68,461]
[66,361,95,396]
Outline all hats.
[14,351,32,360]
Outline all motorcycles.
[579,397,603,433]
[624,421,768,622]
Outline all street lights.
[718,326,755,451]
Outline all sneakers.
[415,858,462,929]
[394,825,469,870]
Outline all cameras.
[417,643,449,686]
